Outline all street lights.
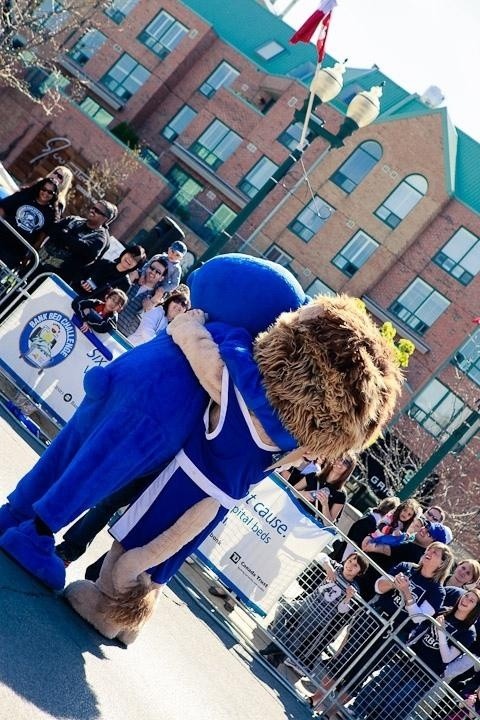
[181,58,387,288]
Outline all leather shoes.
[209,586,227,598]
[225,603,233,611]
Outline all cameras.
[82,277,98,291]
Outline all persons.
[55,475,156,581]
[61,294,406,646]
[189,453,480,720]
[0,166,192,415]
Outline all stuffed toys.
[0,252,310,588]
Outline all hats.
[426,519,453,544]
[170,241,187,257]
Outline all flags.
[288,0,335,64]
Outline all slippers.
[305,697,316,708]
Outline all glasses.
[173,297,187,308]
[425,524,431,538]
[150,265,161,275]
[52,170,63,180]
[91,206,109,219]
[340,457,351,469]
[40,186,54,195]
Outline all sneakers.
[187,556,195,565]
[259,648,278,668]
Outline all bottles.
[309,487,330,500]
